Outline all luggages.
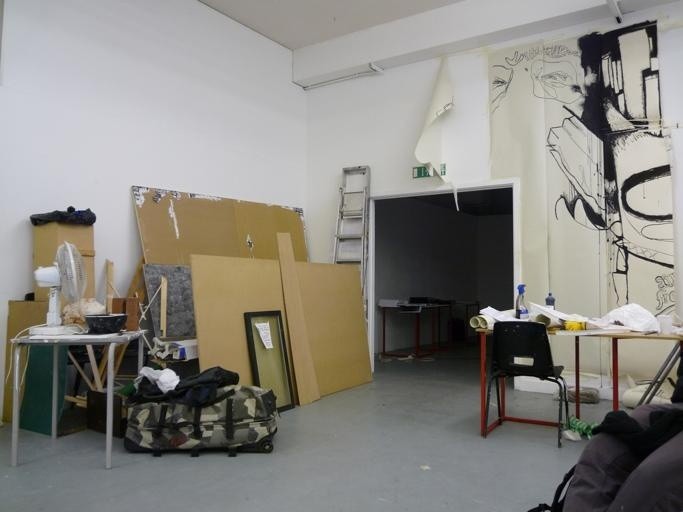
[124,384,278,457]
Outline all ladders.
[333,166,371,332]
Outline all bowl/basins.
[84,313,128,335]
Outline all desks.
[9,329,148,469]
[378,300,480,358]
[475,324,683,438]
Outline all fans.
[29,240,88,335]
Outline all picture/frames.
[243,310,295,414]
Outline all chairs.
[484,321,571,448]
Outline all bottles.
[545,292,556,310]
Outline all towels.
[133,366,182,393]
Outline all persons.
[562,339,683,511]
